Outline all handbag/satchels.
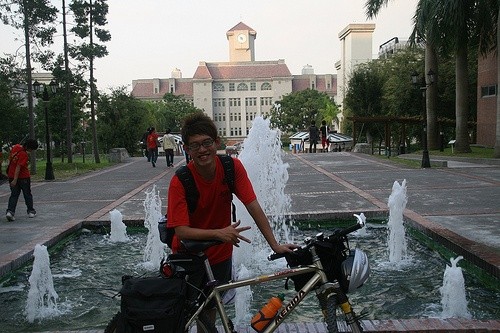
[6,164,10,173]
[154,139,161,147]
[326,135,330,140]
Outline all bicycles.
[105,223,364,333]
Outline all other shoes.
[152,161,155,167]
[6,211,16,222]
[28,210,37,217]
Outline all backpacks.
[158,155,235,250]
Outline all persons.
[6,138,38,222]
[146,127,161,167]
[307,120,319,154]
[319,120,330,153]
[163,127,176,169]
[166,111,300,333]
[141,127,152,162]
[183,142,192,164]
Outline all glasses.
[186,137,214,151]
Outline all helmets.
[343,246,370,293]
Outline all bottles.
[250,293,285,333]
